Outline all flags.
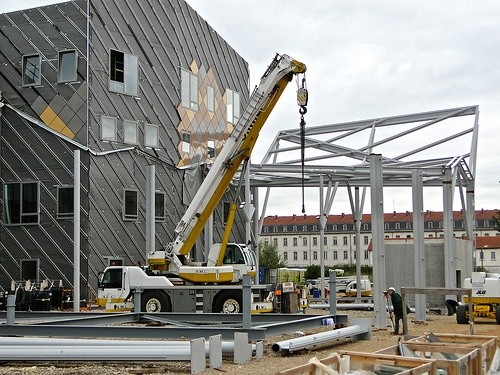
[367,239,372,251]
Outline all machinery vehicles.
[94,52,310,314]
[456,270,500,325]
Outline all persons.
[446,299,462,316]
[387,286,410,335]
[226,250,236,263]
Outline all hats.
[389,287,395,292]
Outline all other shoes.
[391,331,398,335]
[400,332,404,335]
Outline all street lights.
[481,244,488,271]
